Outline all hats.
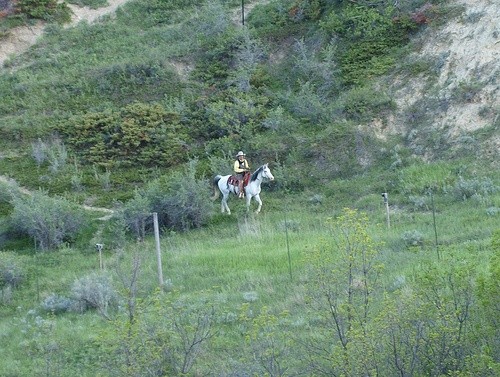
[236,151,246,157]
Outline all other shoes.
[239,193,244,198]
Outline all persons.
[234,151,250,198]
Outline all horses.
[210,162,274,216]
